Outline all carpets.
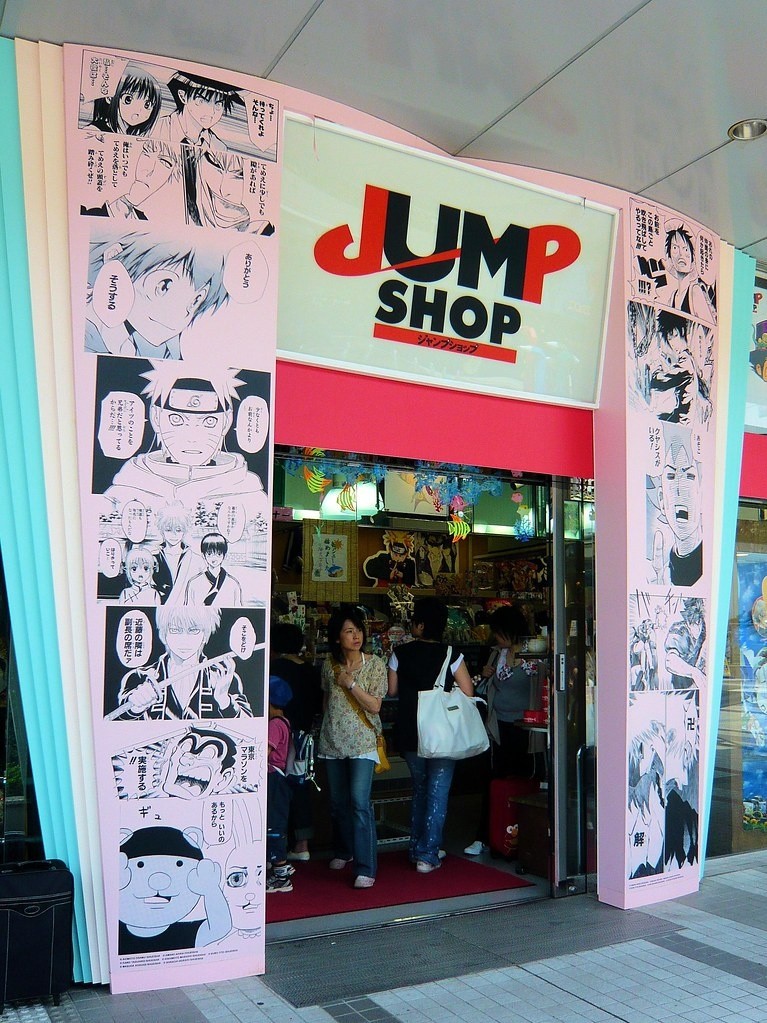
[266,848,539,923]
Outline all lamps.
[318,470,384,523]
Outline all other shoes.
[286,850,310,860]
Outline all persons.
[386,599,474,872]
[463,603,549,856]
[266,624,321,892]
[316,607,387,888]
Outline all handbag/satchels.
[417,645,491,759]
[476,649,499,694]
[373,734,391,774]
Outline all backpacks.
[267,715,321,792]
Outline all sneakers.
[438,850,446,858]
[416,859,441,873]
[464,841,486,855]
[331,856,353,869]
[266,859,296,877]
[354,875,375,887]
[266,875,293,893]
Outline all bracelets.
[350,680,356,690]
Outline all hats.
[269,676,293,706]
